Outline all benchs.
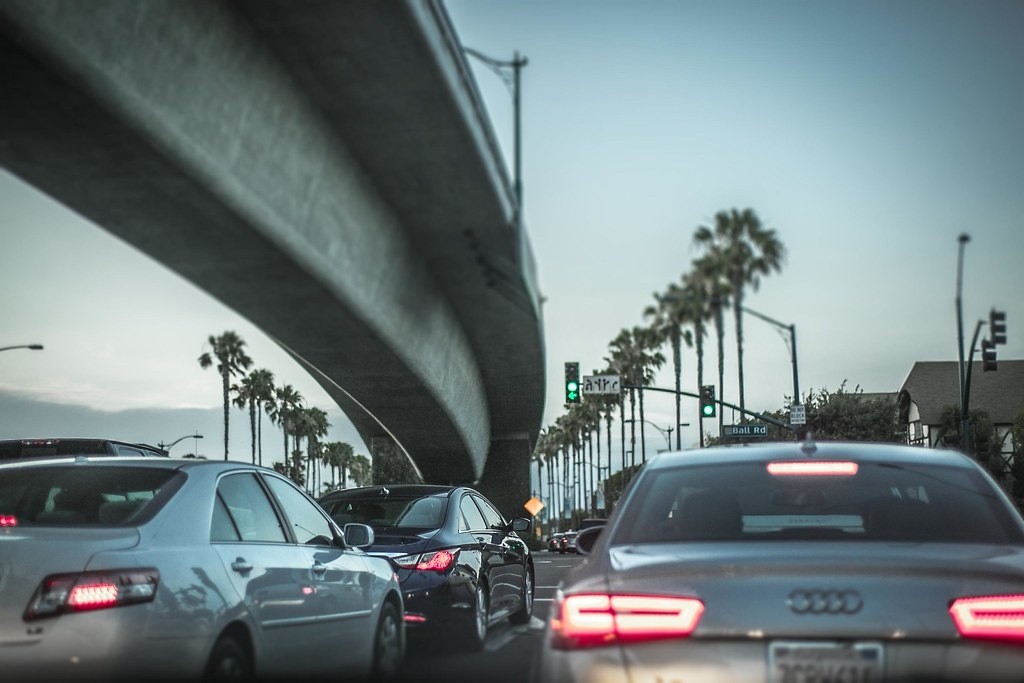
[6,497,149,523]
[328,510,439,529]
[678,490,942,539]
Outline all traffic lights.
[699,384,716,417]
[988,305,1007,344]
[564,361,580,403]
[981,338,999,372]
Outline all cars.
[0,436,534,683]
[540,439,1024,683]
[547,531,580,554]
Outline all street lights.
[157,433,203,453]
[546,482,574,497]
[955,232,974,455]
[623,418,689,449]
[572,460,609,482]
